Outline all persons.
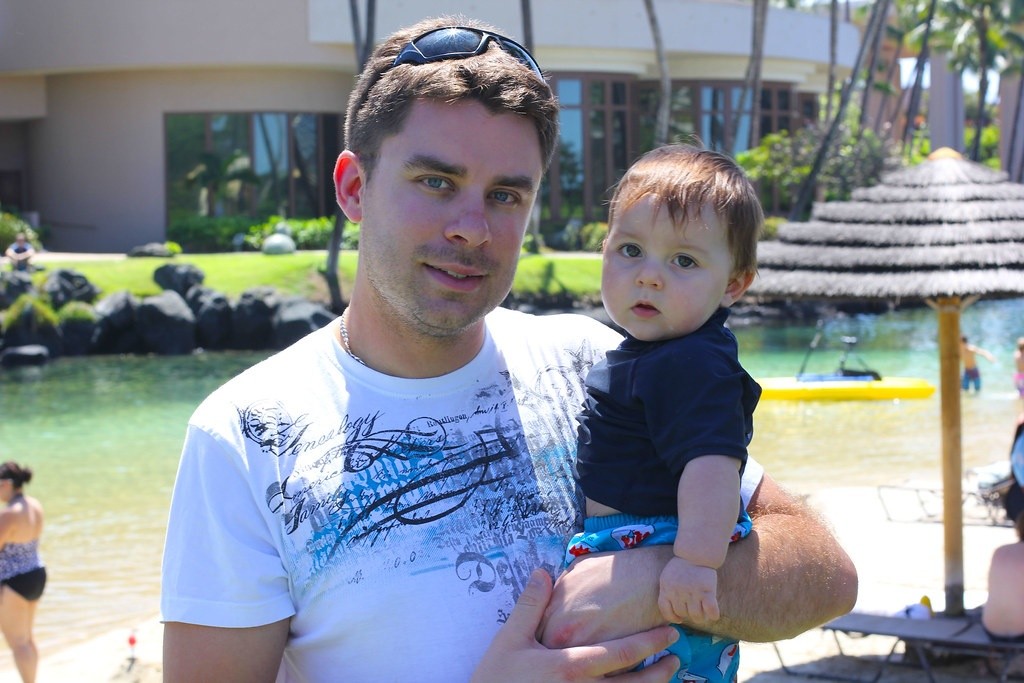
[1015,337,1024,427]
[4,232,35,275]
[560,143,761,683]
[978,420,1024,638]
[958,336,996,394]
[161,16,858,683]
[0,462,46,683]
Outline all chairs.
[772,613,1024,683]
[875,459,1014,523]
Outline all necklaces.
[340,305,367,368]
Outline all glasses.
[355,25,544,113]
